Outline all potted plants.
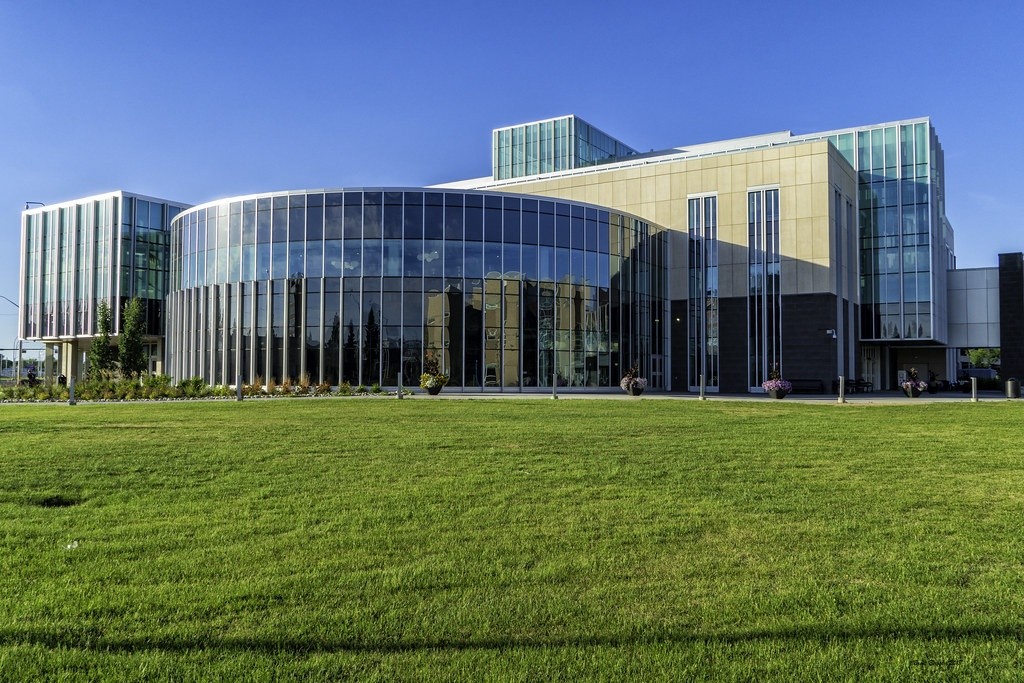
[926,369,940,394]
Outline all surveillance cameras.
[833,335,836,340]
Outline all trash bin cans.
[1003,378,1019,398]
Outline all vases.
[427,386,441,396]
[627,388,642,395]
[902,388,922,398]
[768,389,787,399]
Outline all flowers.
[761,363,793,394]
[620,369,647,392]
[899,367,928,391]
[420,373,449,388]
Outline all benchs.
[834,379,874,393]
